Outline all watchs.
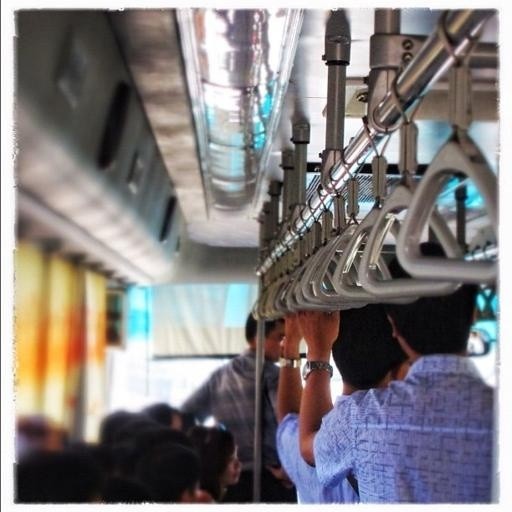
[302,361,333,380]
[275,356,301,369]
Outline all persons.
[179,312,297,502]
[16,402,242,503]
[296,242,499,503]
[276,305,411,504]
